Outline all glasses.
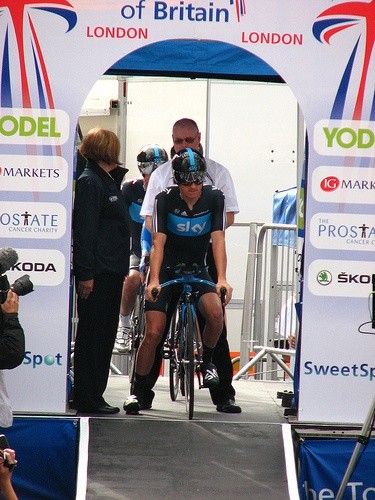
[181,181,203,186]
[172,135,197,144]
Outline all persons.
[72,129,132,418]
[0,448,19,500]
[123,147,233,412]
[0,247,25,432]
[112,144,202,356]
[139,118,242,413]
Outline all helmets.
[137,145,169,162]
[173,147,206,171]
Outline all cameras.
[0,273,35,303]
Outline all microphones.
[0,246,18,274]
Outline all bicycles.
[109,257,227,421]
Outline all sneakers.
[123,395,140,412]
[201,361,219,386]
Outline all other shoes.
[115,324,133,353]
[141,389,155,408]
[215,398,242,413]
[78,406,119,415]
